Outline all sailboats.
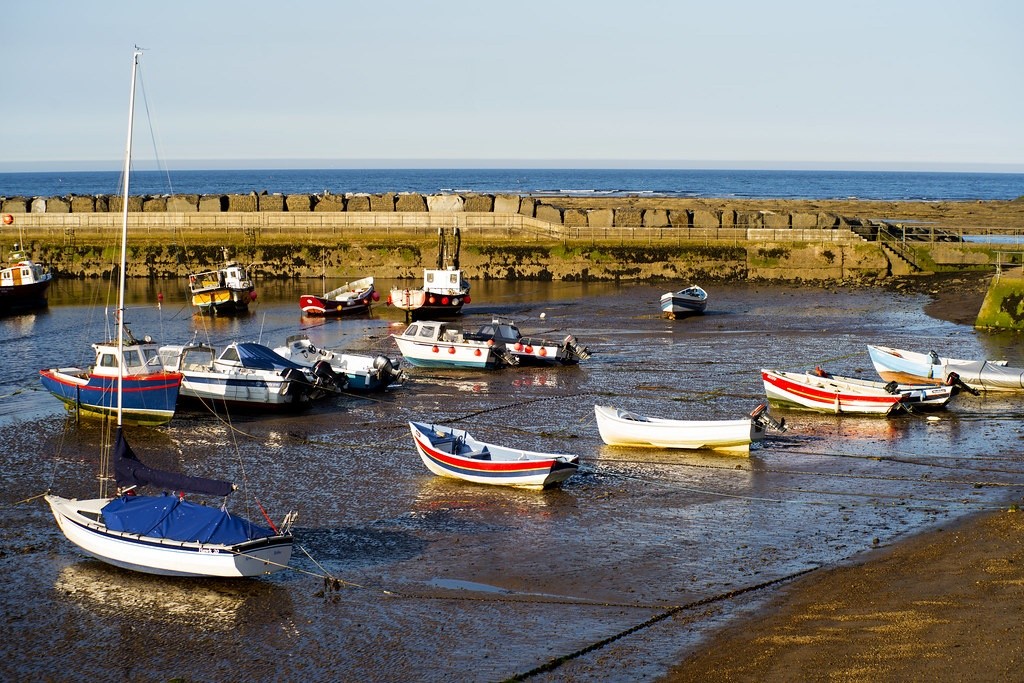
[44,45,301,577]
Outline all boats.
[39,314,183,426]
[594,403,787,452]
[0,243,52,297]
[760,366,962,417]
[867,344,1024,392]
[158,338,342,407]
[390,318,592,368]
[298,277,380,318]
[188,261,258,316]
[387,227,472,323]
[660,284,708,321]
[409,421,580,490]
[273,334,403,394]
